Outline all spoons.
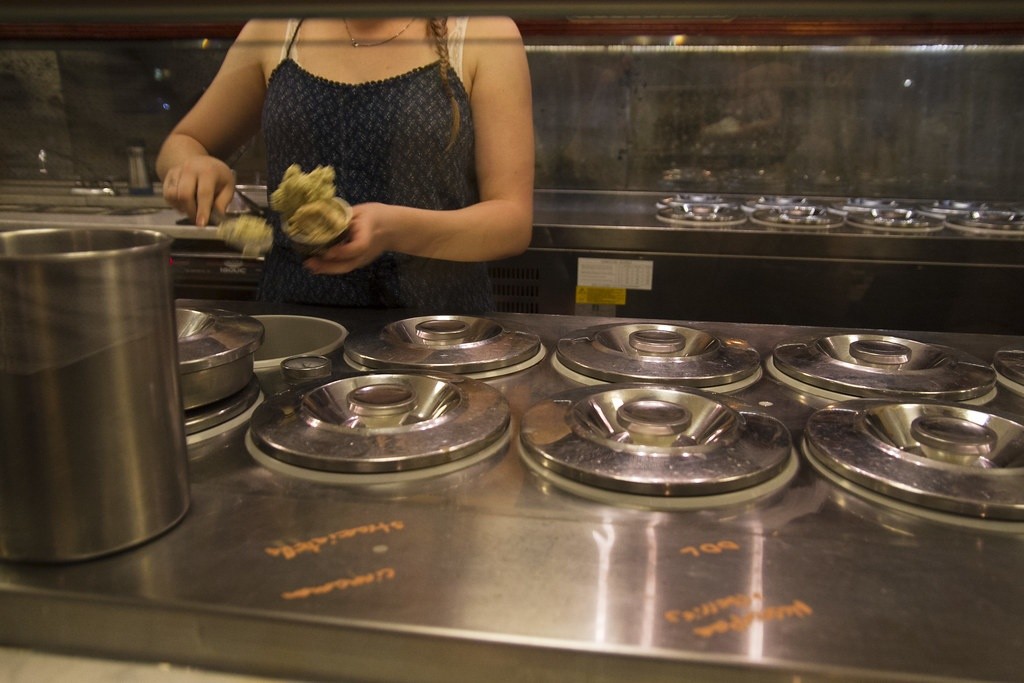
[207,201,273,255]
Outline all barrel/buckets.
[0,225,197,566]
[990,363,1024,397]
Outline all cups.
[126,145,154,195]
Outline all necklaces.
[343,17,416,47]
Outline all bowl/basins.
[243,314,350,379]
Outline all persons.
[155,17,535,313]
